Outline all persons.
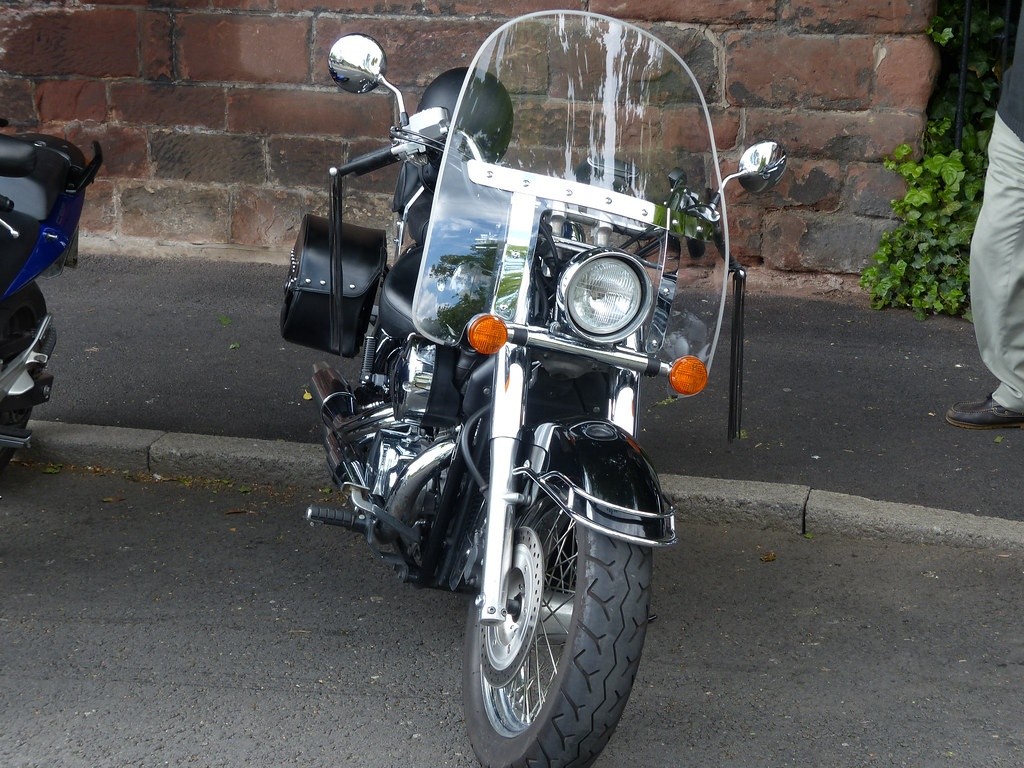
[945,7,1024,429]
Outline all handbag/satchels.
[280,170,385,358]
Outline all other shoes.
[945,393,1023,430]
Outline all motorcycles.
[1,115,104,481]
[273,9,790,768]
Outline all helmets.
[416,66,514,165]
